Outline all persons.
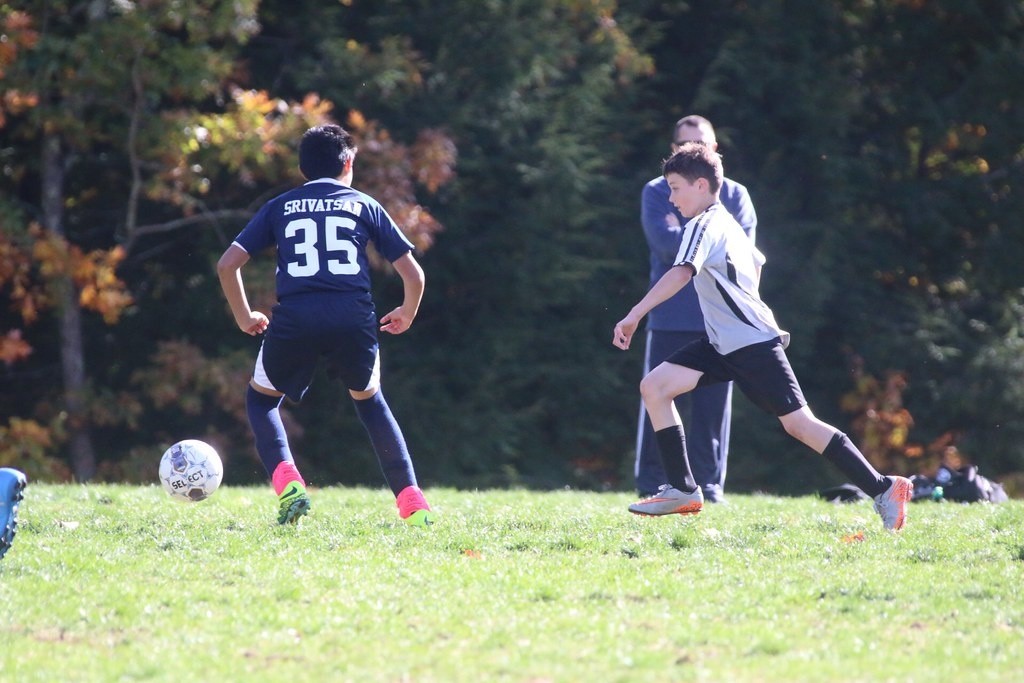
[0,466,28,561]
[612,142,912,537]
[634,115,758,505]
[216,124,439,529]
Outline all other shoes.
[0,467,27,561]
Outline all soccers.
[158,438,225,501]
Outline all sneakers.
[628,484,703,516]
[872,475,914,530]
[272,480,310,527]
[403,509,436,530]
